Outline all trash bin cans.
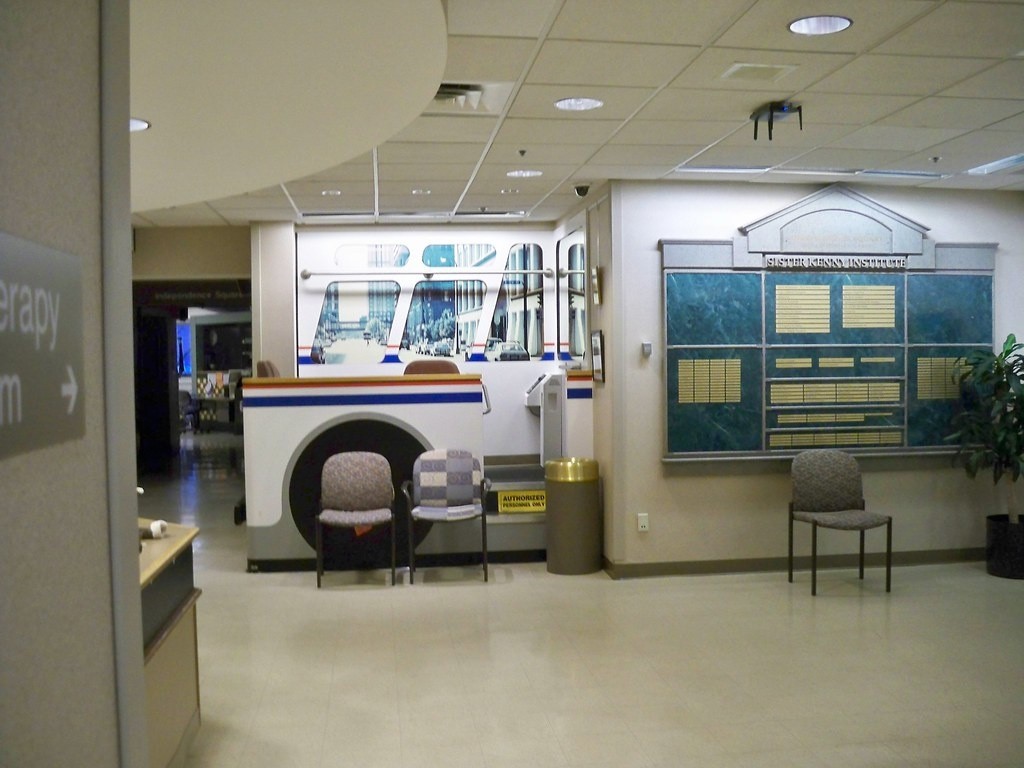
[543,458,605,576]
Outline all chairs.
[178,390,199,433]
[787,448,893,597]
[402,448,491,584]
[315,452,395,588]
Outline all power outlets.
[637,513,649,531]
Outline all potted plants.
[951,333,1024,579]
